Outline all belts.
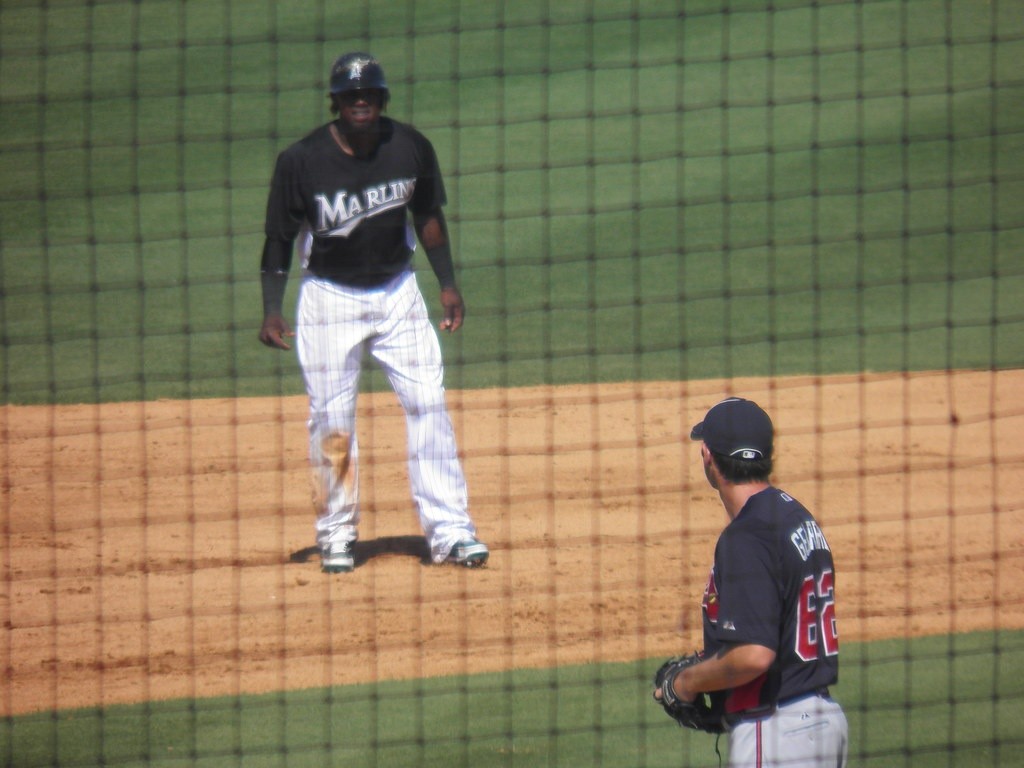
[779,686,831,708]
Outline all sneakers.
[320,537,355,573]
[446,537,490,568]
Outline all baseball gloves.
[653,652,728,734]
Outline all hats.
[690,396,773,461]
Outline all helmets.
[327,52,389,115]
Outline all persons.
[653,397,849,768]
[257,53,488,574]
